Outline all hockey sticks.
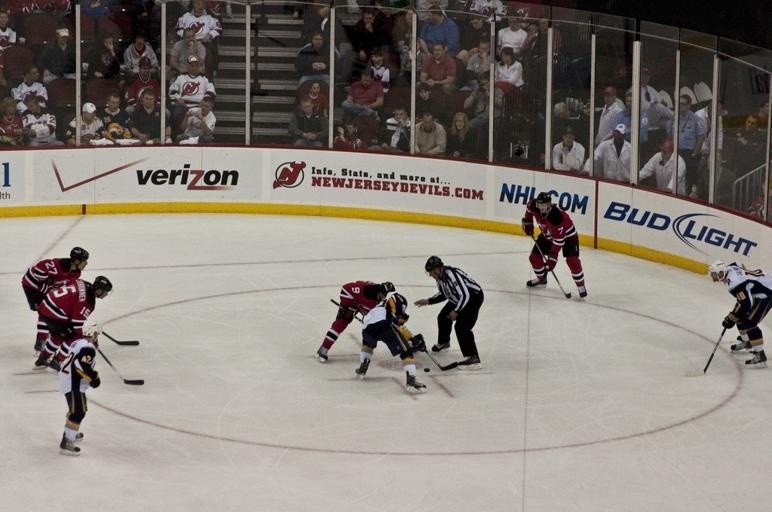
[97,348,144,385]
[424,349,457,371]
[685,327,727,376]
[102,332,139,344]
[531,235,571,298]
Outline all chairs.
[0,0,174,141]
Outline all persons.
[414,256,484,364]
[356,291,426,389]
[318,280,398,360]
[0,0,232,146]
[288,0,772,225]
[520,192,588,298]
[34,275,113,369]
[709,261,772,365]
[61,321,102,451]
[21,247,89,311]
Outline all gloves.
[344,307,358,323]
[89,378,100,388]
[722,312,739,329]
[545,257,557,271]
[524,222,534,236]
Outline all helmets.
[425,256,443,272]
[82,319,103,337]
[710,260,728,285]
[380,281,395,297]
[95,276,112,291]
[70,247,88,261]
[536,192,551,209]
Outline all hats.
[56,28,71,37]
[561,126,575,136]
[602,85,616,96]
[187,54,201,63]
[82,101,96,114]
[615,123,627,136]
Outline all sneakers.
[63,431,83,439]
[432,342,450,352]
[578,286,587,297]
[317,345,329,360]
[34,355,52,366]
[355,358,370,376]
[34,340,47,352]
[527,278,547,287]
[49,356,69,374]
[745,351,767,364]
[406,371,426,389]
[458,356,480,366]
[60,437,80,452]
[731,341,752,350]
